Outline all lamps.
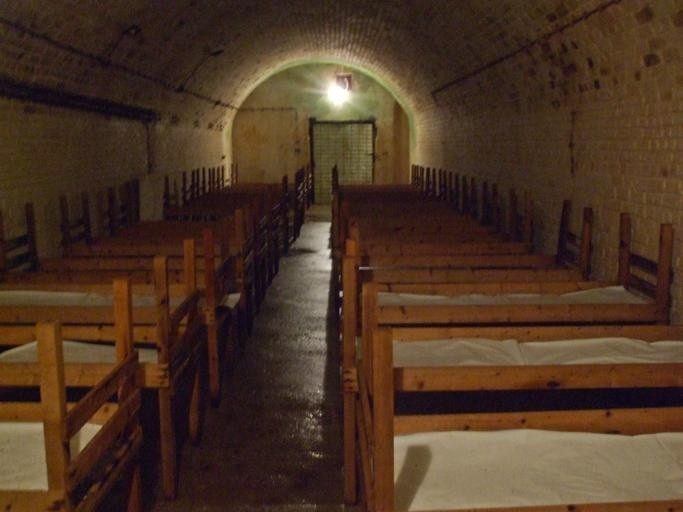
[328,75,353,106]
